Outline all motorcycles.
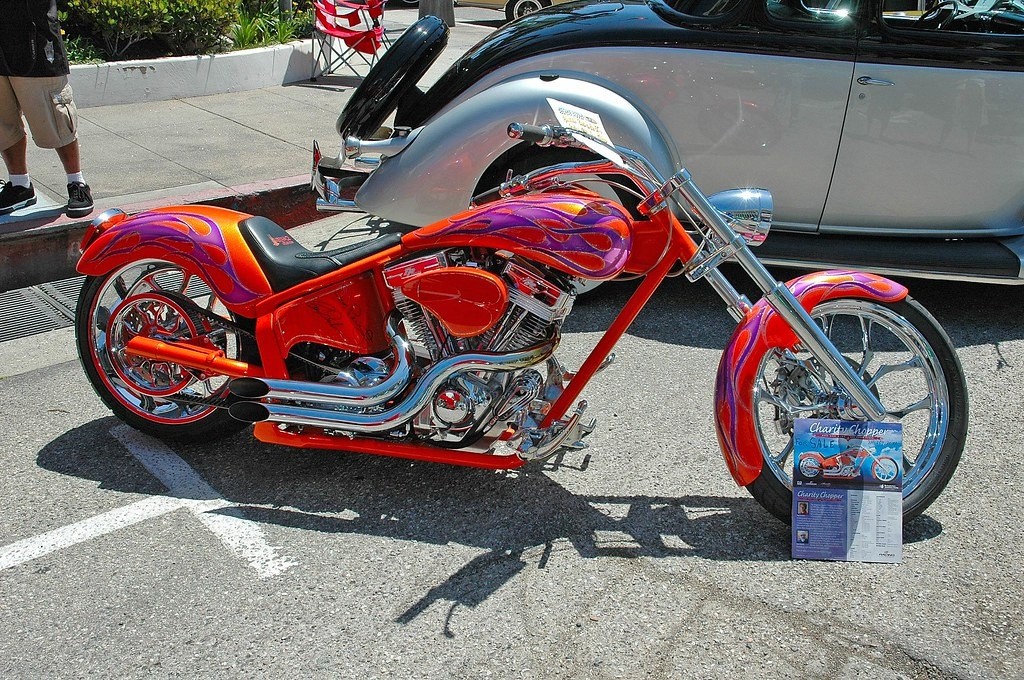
[798,445,899,482]
[76,122,969,533]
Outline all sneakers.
[0,179,37,215]
[66,181,95,213]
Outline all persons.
[0,0,94,218]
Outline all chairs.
[310,0,391,82]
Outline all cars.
[309,0,1024,294]
